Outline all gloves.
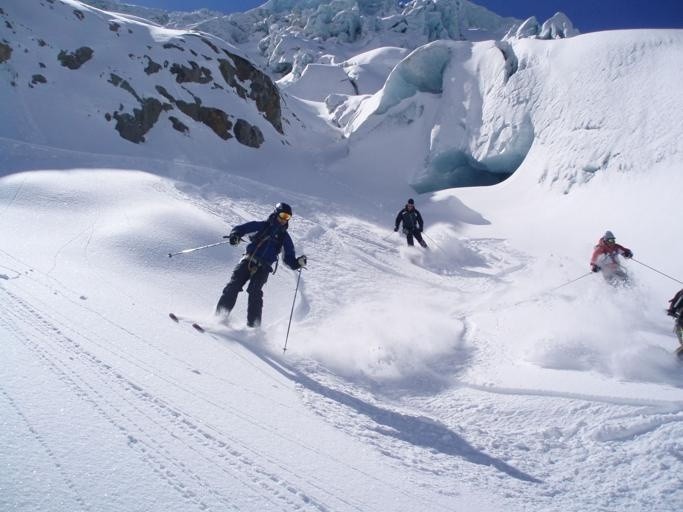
[230,231,239,245]
[593,265,600,272]
[297,255,306,267]
[625,251,633,258]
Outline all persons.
[591,230,633,285]
[214,202,308,330]
[394,198,427,247]
[665,290,683,358]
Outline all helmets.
[408,199,414,204]
[603,231,614,242]
[274,203,292,225]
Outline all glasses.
[279,212,291,220]
